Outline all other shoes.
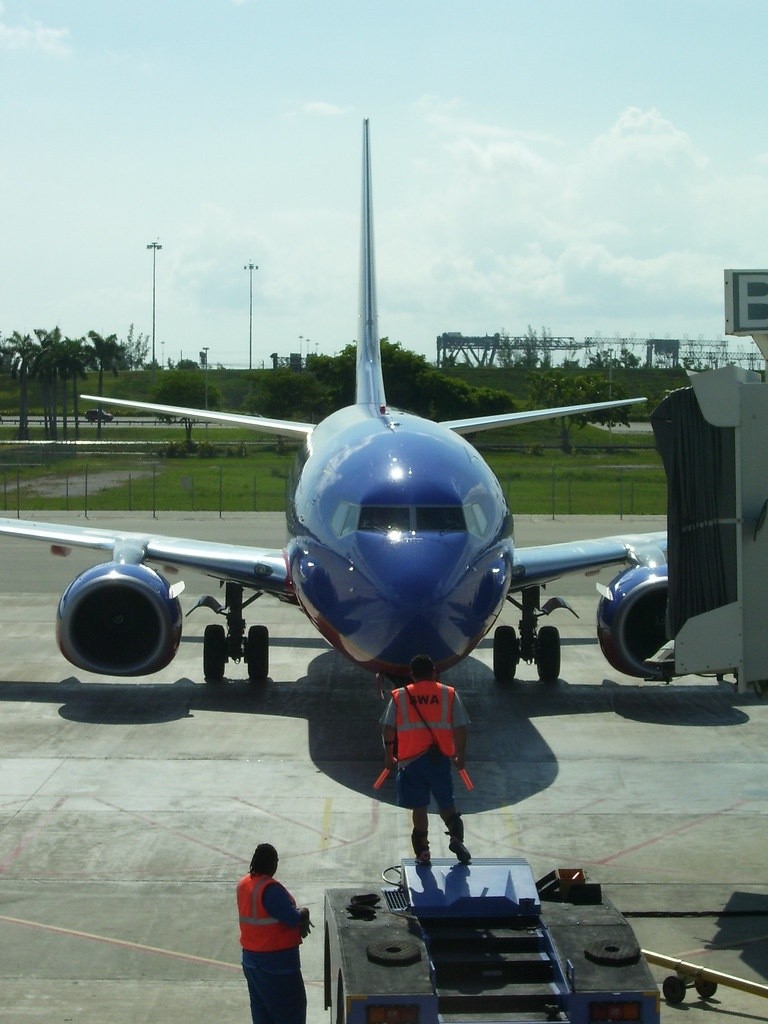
[448,838,471,863]
[415,850,431,865]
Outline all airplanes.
[1,118,691,687]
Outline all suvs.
[85,408,114,422]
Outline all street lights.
[299,336,319,355]
[202,347,210,410]
[243,259,259,371]
[607,349,616,444]
[146,240,164,387]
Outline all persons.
[236,844,309,1024]
[380,654,471,864]
